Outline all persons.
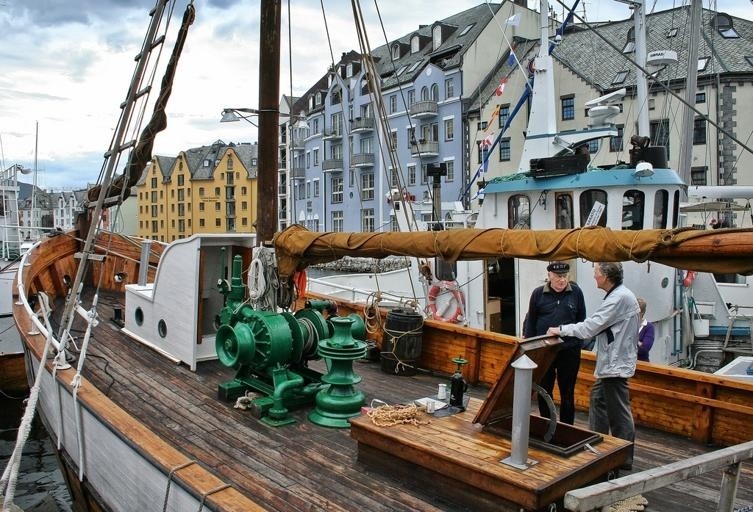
[523,262,586,428]
[547,259,639,470]
[634,297,655,363]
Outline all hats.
[546,263,568,273]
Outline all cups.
[437,383,448,400]
[426,399,436,414]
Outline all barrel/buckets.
[382,310,425,376]
[691,296,710,339]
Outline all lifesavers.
[682,270,697,287]
[429,281,465,324]
